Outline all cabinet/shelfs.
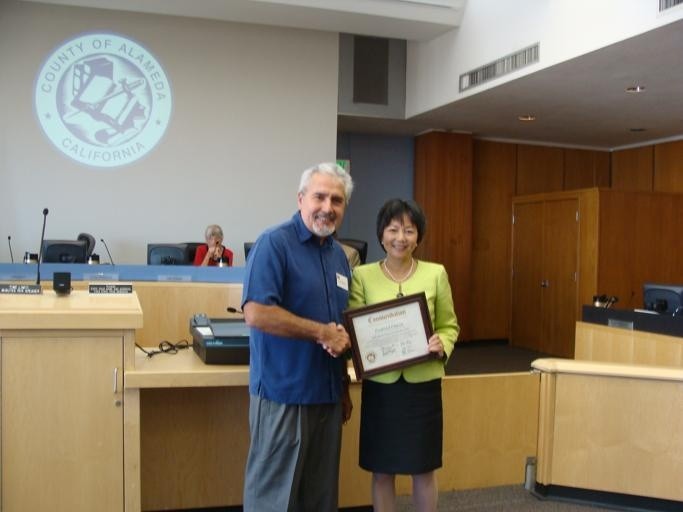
[0,315,142,512]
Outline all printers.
[189,313,250,365]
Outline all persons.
[238,162,354,512]
[192,224,233,266]
[313,195,461,511]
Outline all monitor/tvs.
[244,242,254,260]
[642,283,683,315]
[147,243,189,265]
[42,239,87,263]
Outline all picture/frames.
[342,292,439,382]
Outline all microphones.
[8,236,14,263]
[227,307,244,315]
[36,208,49,284]
[100,239,113,264]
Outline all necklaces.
[382,256,414,284]
